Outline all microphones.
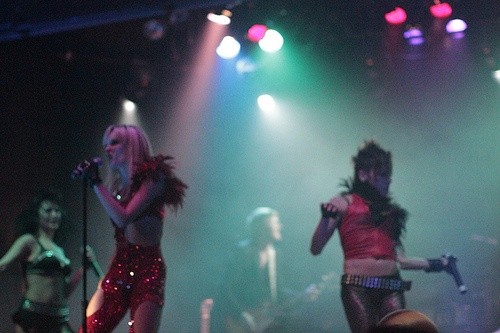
[80,245,103,278]
[73,158,103,178]
[446,254,468,294]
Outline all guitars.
[224,265,342,333]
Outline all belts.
[19,299,70,316]
[341,275,412,291]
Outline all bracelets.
[425,259,442,272]
[90,177,102,189]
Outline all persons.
[79,125,188,333]
[0,196,97,333]
[310,139,458,333]
[199,207,319,333]
